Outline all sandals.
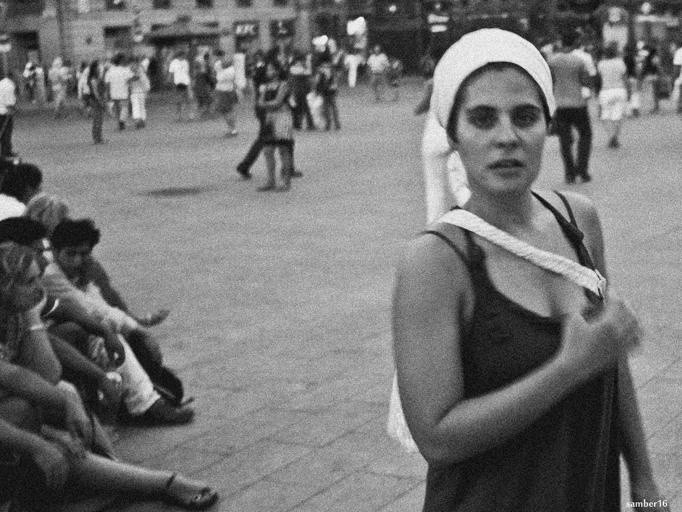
[160,469,219,511]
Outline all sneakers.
[234,165,302,194]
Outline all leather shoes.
[119,395,194,424]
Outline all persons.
[381,22,670,512]
